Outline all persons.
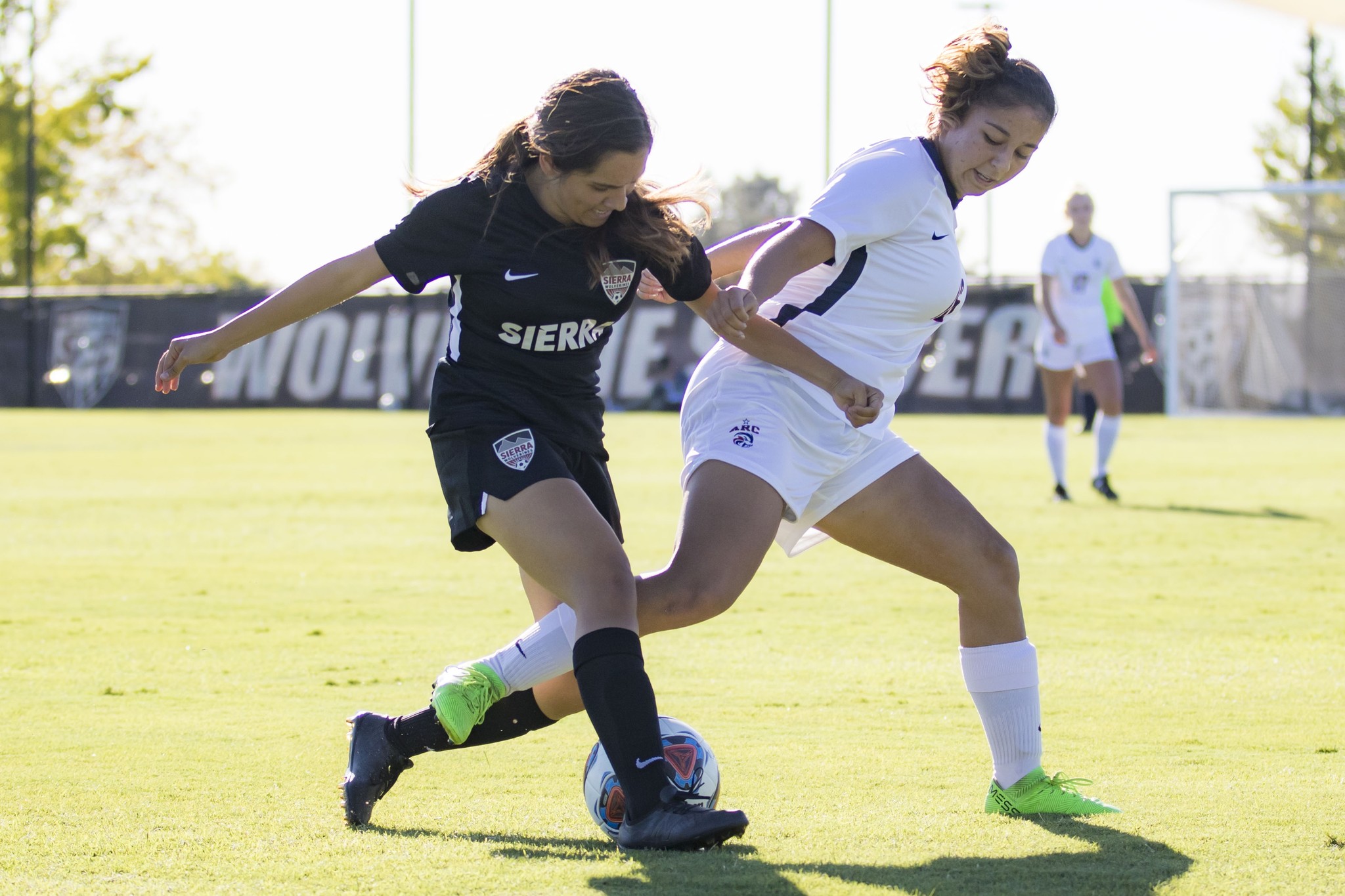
[430,28,1117,819]
[155,69,885,857]
[1030,192,1160,501]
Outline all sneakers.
[429,660,507,746]
[338,710,414,830]
[1091,475,1117,500]
[984,766,1123,816]
[1055,485,1070,501]
[618,766,750,852]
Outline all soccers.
[580,718,723,845]
